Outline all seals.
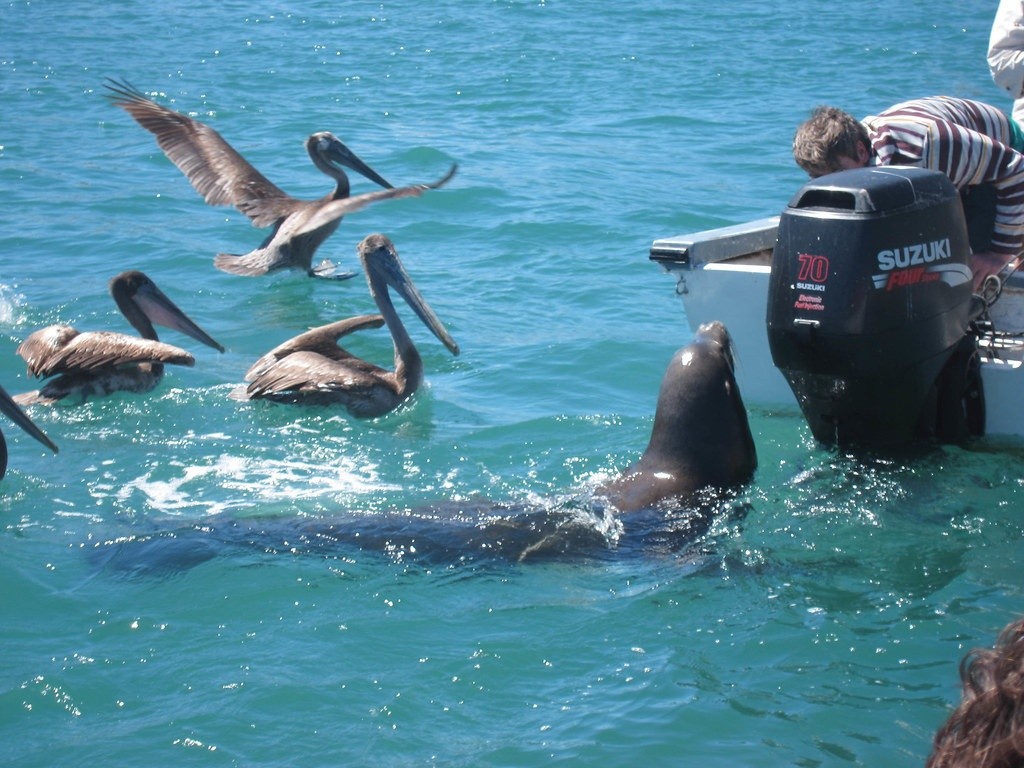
[590,321,757,514]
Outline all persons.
[984,0,1024,128]
[791,97,1024,296]
[927,618,1024,768]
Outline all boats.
[650,166,1024,471]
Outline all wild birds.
[12,271,225,407]
[0,386,58,480]
[101,76,457,281]
[228,235,460,417]
